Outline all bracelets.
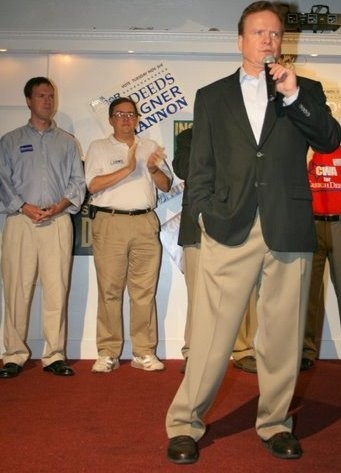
[153,168,159,174]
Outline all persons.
[85,98,173,373]
[298,145,341,368]
[173,129,262,374]
[165,0,341,465]
[0,77,86,379]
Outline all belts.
[314,215,339,221]
[91,204,151,215]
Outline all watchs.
[18,202,26,212]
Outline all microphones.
[263,55,276,101]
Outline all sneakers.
[131,354,164,371]
[91,356,120,373]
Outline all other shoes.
[233,355,257,373]
[180,360,187,373]
[300,358,311,371]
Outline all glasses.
[112,111,137,120]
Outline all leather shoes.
[0,363,22,378]
[167,435,199,464]
[260,431,302,459]
[43,360,72,375]
